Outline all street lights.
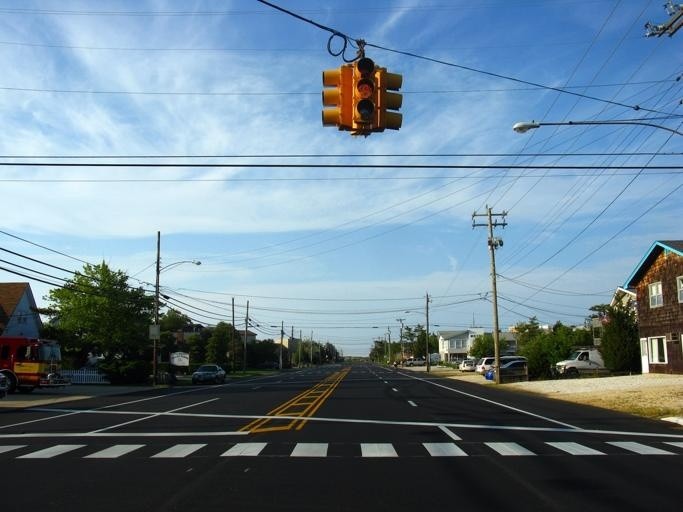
[150,258,201,387]
[241,322,259,374]
[404,309,430,372]
[512,113,683,139]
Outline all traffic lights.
[350,57,380,127]
[372,64,404,131]
[321,64,355,132]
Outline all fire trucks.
[0,333,73,394]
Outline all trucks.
[553,348,604,379]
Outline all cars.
[191,362,227,387]
[0,372,8,397]
[404,357,425,366]
[458,355,529,382]
